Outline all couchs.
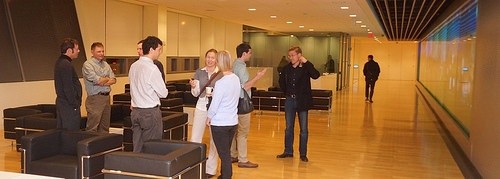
[3,79,333,179]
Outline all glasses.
[288,54,299,58]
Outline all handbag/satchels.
[367,72,378,83]
[193,97,199,106]
[237,87,255,114]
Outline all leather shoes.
[277,153,293,158]
[238,161,258,168]
[230,157,238,163]
[300,156,308,162]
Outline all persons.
[128,35,169,153]
[279,56,289,66]
[188,49,224,179]
[277,46,321,162]
[324,55,335,73]
[204,50,242,179]
[136,39,166,85]
[362,54,380,103]
[53,38,83,127]
[230,42,268,169]
[81,42,117,135]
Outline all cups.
[206,87,214,94]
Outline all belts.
[98,92,109,96]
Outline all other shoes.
[365,99,368,100]
[206,173,214,178]
[370,100,373,103]
